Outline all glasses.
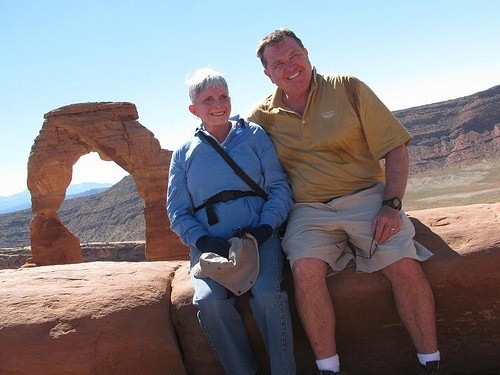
[335,236,378,259]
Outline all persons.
[245,26,444,375]
[165,68,295,375]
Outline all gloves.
[233,224,273,245]
[196,235,231,258]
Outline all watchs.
[379,197,402,211]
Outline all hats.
[192,232,260,296]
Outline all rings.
[391,227,396,232]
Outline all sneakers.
[418,361,442,375]
